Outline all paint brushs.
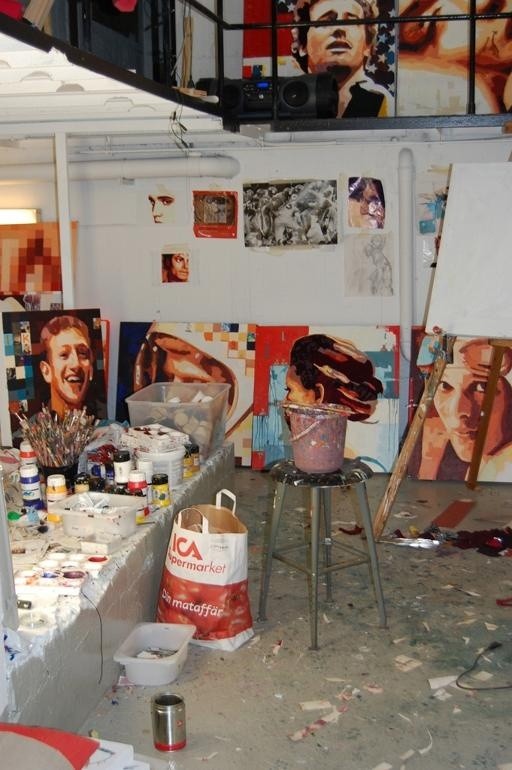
[14,403,100,467]
[280,400,356,414]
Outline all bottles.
[150,693,186,751]
[18,440,171,523]
[183,444,202,475]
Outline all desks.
[4,439,234,735]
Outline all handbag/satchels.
[154,490,255,651]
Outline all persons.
[11,315,106,448]
[282,334,384,458]
[291,0,394,118]
[133,322,252,466]
[148,179,395,298]
[438,335,512,482]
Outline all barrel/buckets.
[285,402,351,475]
[135,446,186,487]
[39,291,62,311]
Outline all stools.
[260,458,386,651]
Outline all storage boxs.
[111,621,197,686]
[125,382,232,459]
